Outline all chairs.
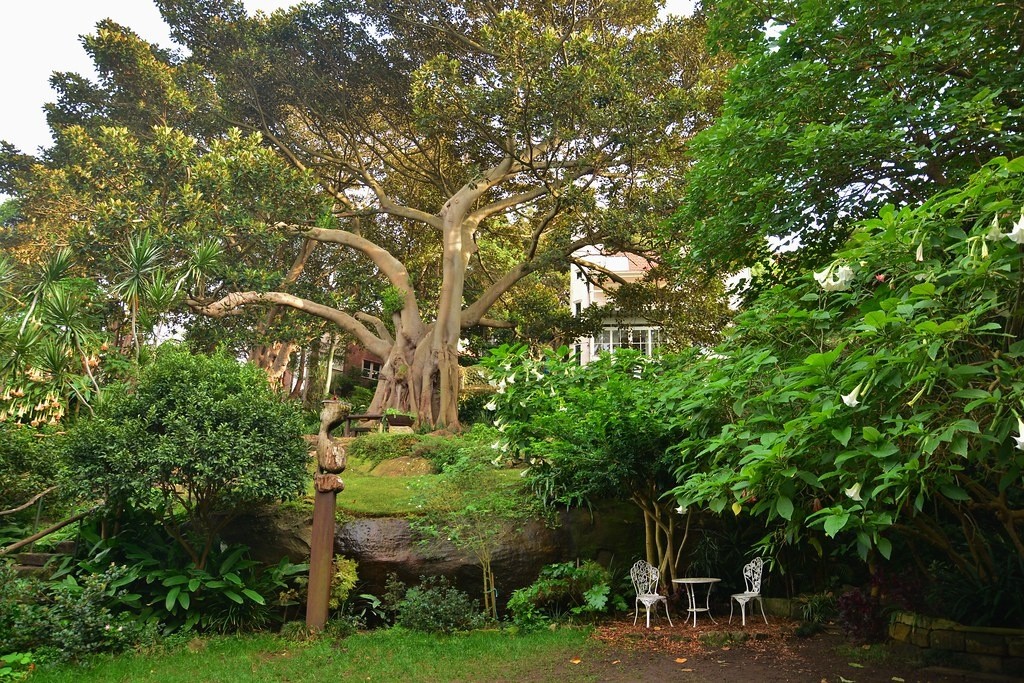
[728,557,768,626]
[630,560,674,629]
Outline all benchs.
[350,427,376,437]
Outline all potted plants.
[382,408,417,427]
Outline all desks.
[672,578,721,628]
[344,415,383,437]
